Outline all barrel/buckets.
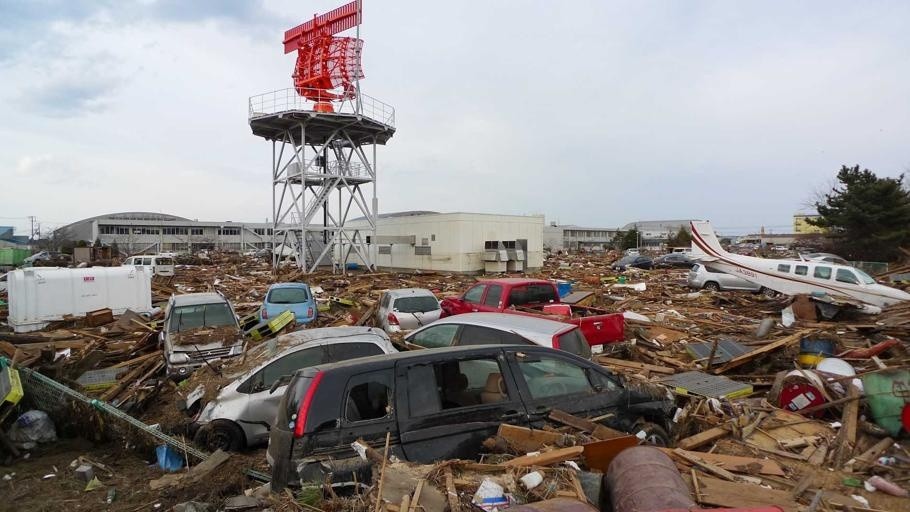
[347,262,358,270]
[604,444,698,512]
[779,368,825,419]
[644,504,785,512]
[816,356,856,395]
[501,496,601,511]
[557,282,572,299]
[542,466,605,512]
[797,333,835,371]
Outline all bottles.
[878,456,894,466]
[105,488,116,504]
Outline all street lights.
[636,231,639,248]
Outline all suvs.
[156,288,247,380]
[687,263,779,296]
[374,288,446,333]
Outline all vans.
[121,255,174,279]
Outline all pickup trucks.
[439,275,623,345]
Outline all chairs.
[480,372,509,404]
[443,372,476,406]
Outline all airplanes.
[691,220,910,315]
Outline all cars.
[258,282,317,326]
[23,249,72,267]
[612,246,695,272]
[805,252,848,265]
[196,248,212,254]
[726,240,788,252]
[243,249,257,256]
